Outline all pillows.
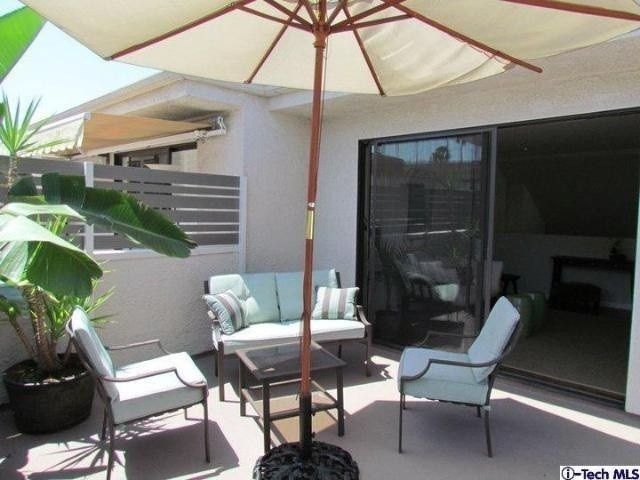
[200,289,249,335]
[470,258,504,295]
[310,285,360,321]
[406,272,440,302]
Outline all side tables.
[500,273,522,295]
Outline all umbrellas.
[16,0,640,457]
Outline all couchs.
[204,269,373,402]
[397,261,480,334]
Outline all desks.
[550,255,634,310]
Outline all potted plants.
[0,87,197,435]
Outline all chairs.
[65,308,210,480]
[396,295,524,457]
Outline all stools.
[522,289,546,329]
[511,293,532,337]
